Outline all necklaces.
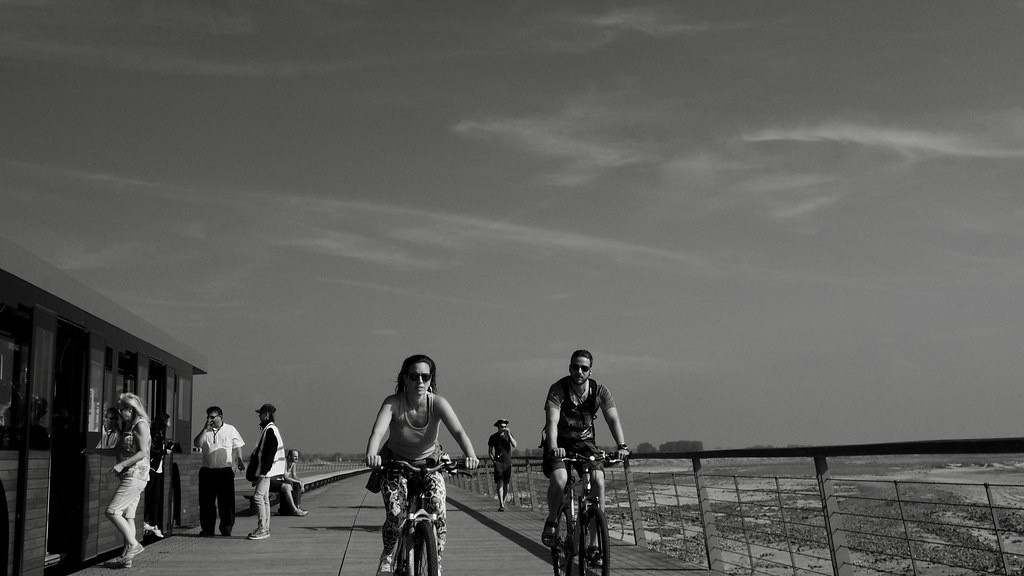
[212,427,221,443]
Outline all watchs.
[618,444,628,450]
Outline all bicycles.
[365,457,473,576]
[550,451,625,576]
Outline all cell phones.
[166,441,174,450]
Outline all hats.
[494,419,509,427]
[255,404,276,414]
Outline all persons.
[488,420,517,511]
[194,406,246,537]
[542,350,629,566]
[270,448,308,516]
[0,380,51,447]
[94,408,121,448]
[366,354,479,576]
[103,394,151,568]
[143,413,171,537]
[247,404,285,540]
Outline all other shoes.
[144,523,155,530]
[221,528,231,536]
[153,529,164,537]
[292,508,309,516]
[199,530,215,537]
[122,543,144,563]
[118,556,132,567]
[498,503,508,511]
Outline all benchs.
[234,467,373,517]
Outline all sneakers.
[247,527,270,539]
[376,552,394,576]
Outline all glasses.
[570,363,591,371]
[106,416,119,421]
[406,371,432,382]
[119,407,131,414]
[499,426,507,429]
[207,415,220,419]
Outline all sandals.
[541,517,558,547]
[586,547,603,565]
